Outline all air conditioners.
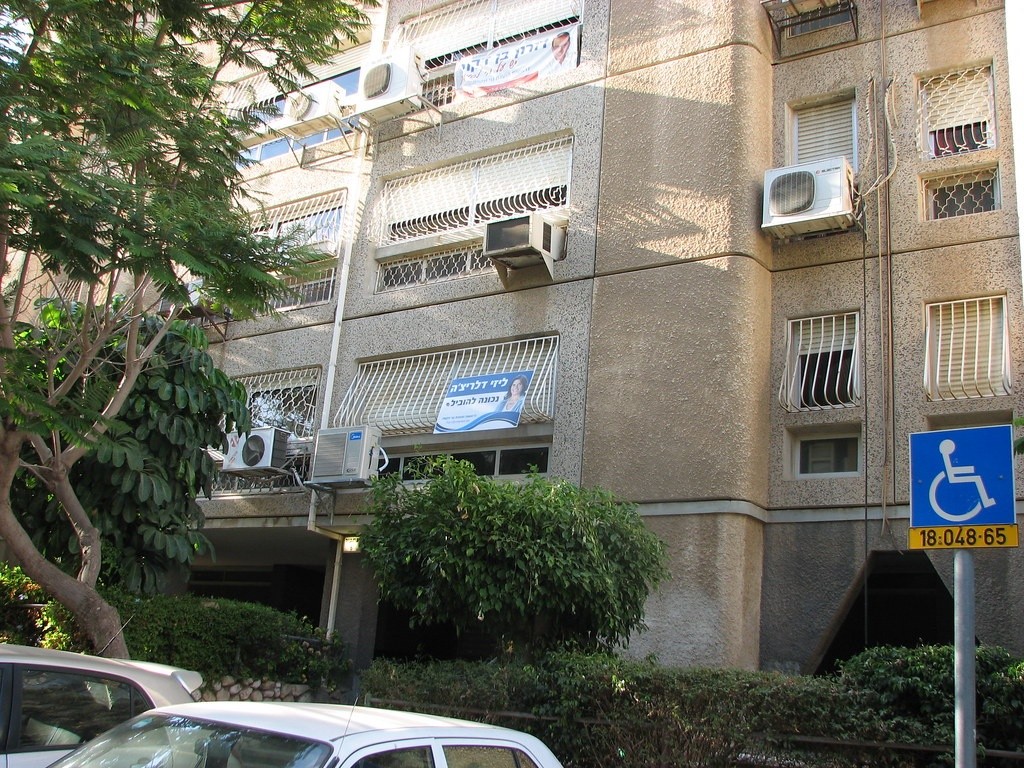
[282,81,346,134]
[782,0,840,17]
[354,48,422,119]
[761,155,854,240]
[219,425,288,472]
[481,213,565,266]
[310,424,381,485]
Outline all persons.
[548,32,576,70]
[496,375,527,413]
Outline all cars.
[0,642,213,768]
[46,701,563,768]
